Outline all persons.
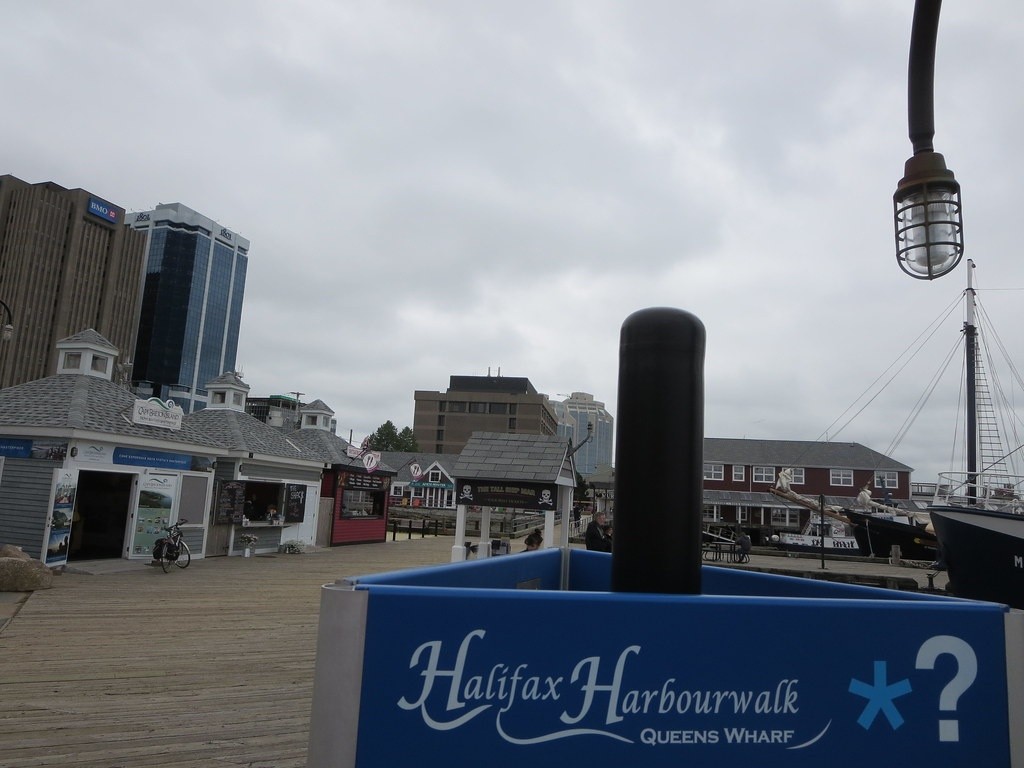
[585,511,613,553]
[520,528,543,552]
[573,505,582,528]
[735,532,751,563]
[245,495,260,521]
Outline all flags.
[361,448,379,473]
[407,458,424,481]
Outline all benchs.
[736,548,750,563]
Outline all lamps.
[212,462,217,469]
[320,473,325,479]
[71,446,78,459]
[240,464,244,472]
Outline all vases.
[285,548,290,554]
[242,549,251,559]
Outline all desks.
[699,548,713,561]
[712,542,736,563]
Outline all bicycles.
[159,519,191,573]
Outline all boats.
[929,437,1024,613]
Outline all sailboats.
[765,257,1024,561]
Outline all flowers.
[240,533,258,549]
[284,538,306,553]
